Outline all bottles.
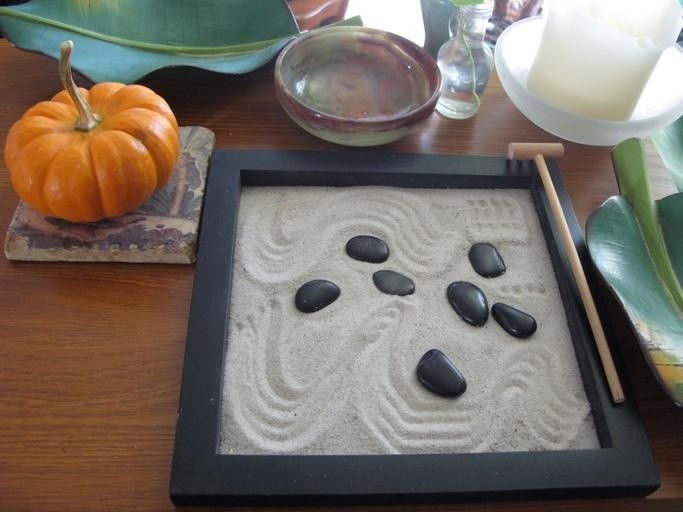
[430,3,496,120]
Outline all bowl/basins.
[274,24,443,148]
[492,14,683,145]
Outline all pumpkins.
[4,40,179,223]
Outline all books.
[2,124,213,266]
